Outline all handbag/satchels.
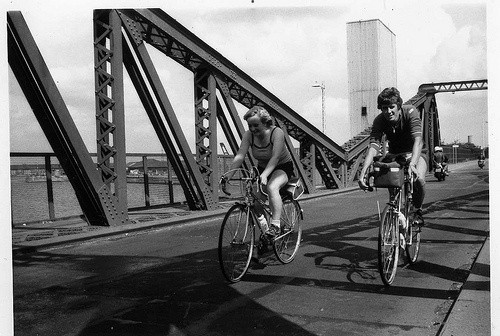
[373,162,406,188]
[287,176,304,200]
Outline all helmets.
[434,146,443,152]
[481,153,483,155]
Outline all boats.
[24,162,70,184]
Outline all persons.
[432,146,448,170]
[477,153,485,162]
[358,87,428,224]
[224,106,293,236]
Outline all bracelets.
[409,162,416,168]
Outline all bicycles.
[212,165,305,284]
[357,168,425,287]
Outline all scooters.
[433,154,450,182]
[477,157,485,169]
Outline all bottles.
[258,213,269,233]
[399,211,407,228]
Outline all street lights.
[311,80,327,137]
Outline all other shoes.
[252,249,275,263]
[265,224,281,239]
[411,205,425,227]
